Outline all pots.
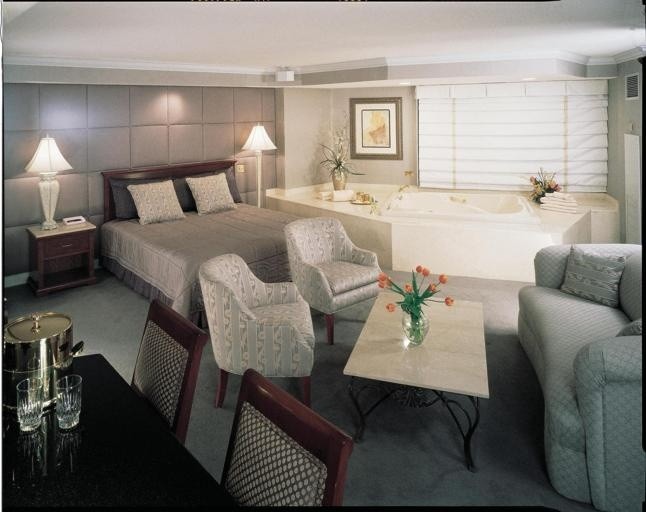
[2,312,84,417]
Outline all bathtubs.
[385,190,542,225]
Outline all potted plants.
[319,144,366,190]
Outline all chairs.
[221,370,356,509]
[132,299,208,443]
[200,252,315,408]
[284,214,382,346]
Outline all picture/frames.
[349,96,403,160]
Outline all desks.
[2,354,246,510]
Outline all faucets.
[398,186,410,193]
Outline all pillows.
[128,179,188,224]
[616,320,643,335]
[562,245,625,307]
[185,171,237,215]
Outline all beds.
[100,160,307,328]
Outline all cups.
[54,375,84,429]
[16,377,45,433]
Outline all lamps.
[26,133,74,230]
[242,123,279,206]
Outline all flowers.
[528,168,562,201]
[379,265,455,311]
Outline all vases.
[400,308,429,345]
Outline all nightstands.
[26,222,97,299]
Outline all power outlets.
[236,163,248,174]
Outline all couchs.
[519,243,646,511]
